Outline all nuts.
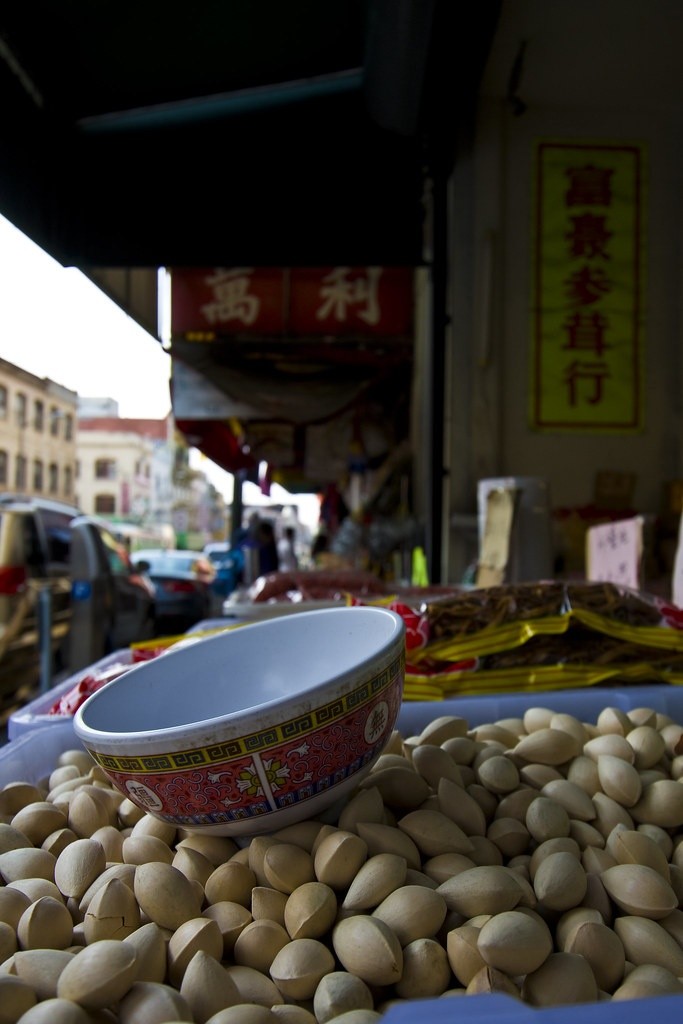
[0,705,683,1024]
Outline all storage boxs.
[0,591,682,1023]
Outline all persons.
[232,511,298,586]
[310,515,332,560]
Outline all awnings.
[1,0,505,493]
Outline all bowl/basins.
[71,605,409,839]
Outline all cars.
[1,489,241,740]
